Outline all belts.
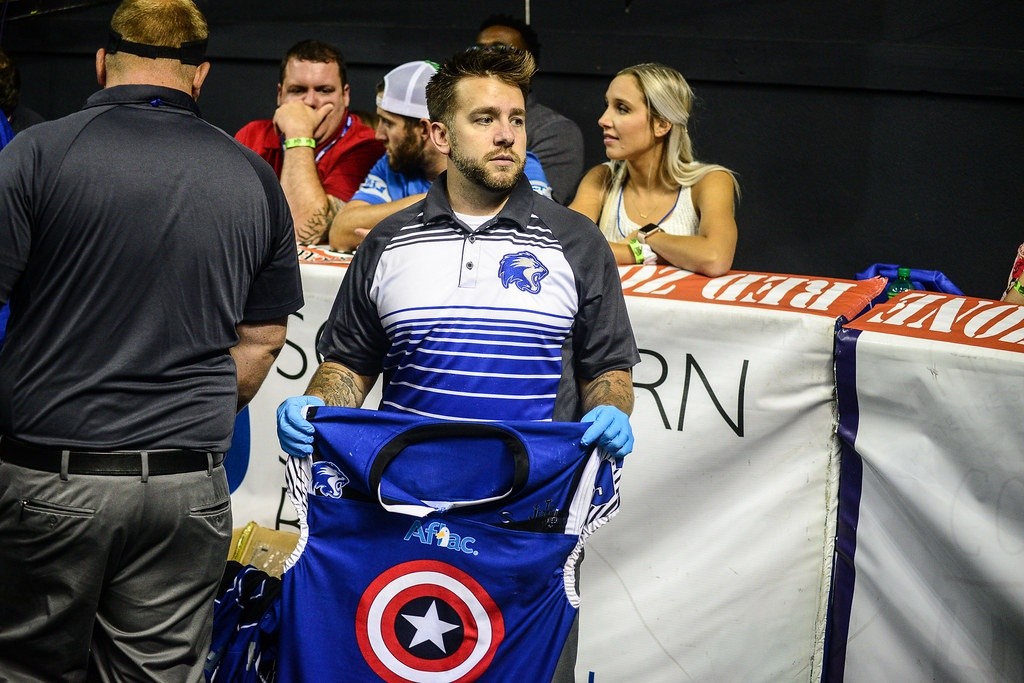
[0,434,225,477]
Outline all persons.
[0,51,47,137]
[1000,243,1024,305]
[275,43,643,683]
[232,18,738,278]
[0,0,305,683]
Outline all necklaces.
[628,187,665,219]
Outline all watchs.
[637,223,665,244]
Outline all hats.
[376,59,445,120]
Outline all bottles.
[887,267,914,301]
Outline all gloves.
[579,403,634,460]
[276,395,325,459]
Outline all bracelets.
[1013,279,1024,297]
[629,238,647,264]
[285,137,316,149]
